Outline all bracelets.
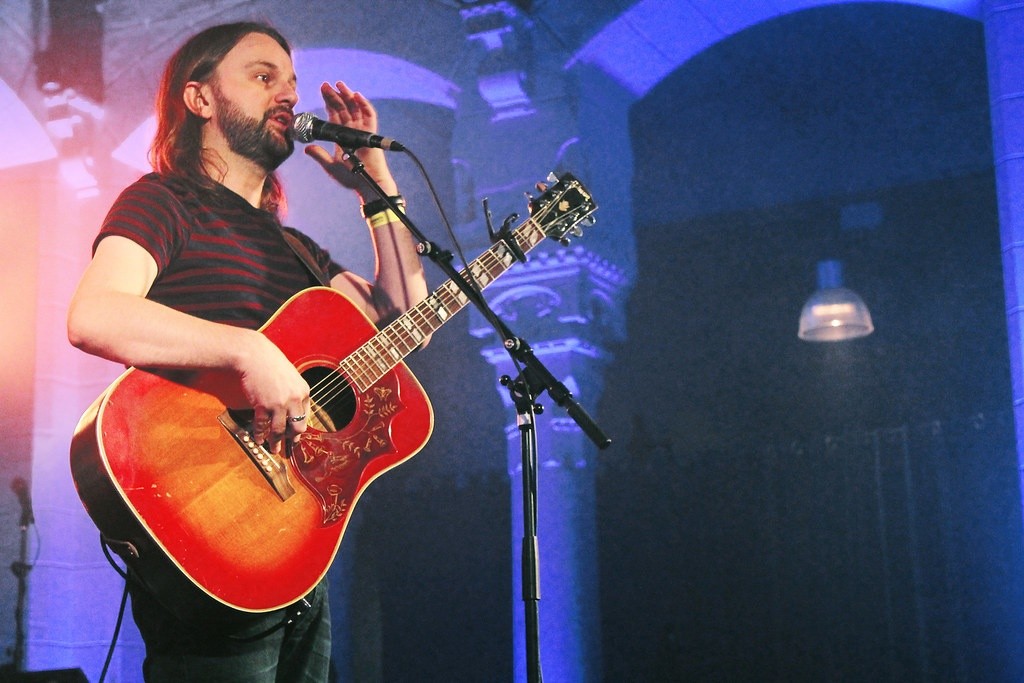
[359,191,407,231]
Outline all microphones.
[11,477,36,523]
[288,112,407,152]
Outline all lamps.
[798,261,874,342]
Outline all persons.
[66,19,434,683]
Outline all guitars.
[69,171,599,634]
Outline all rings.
[286,411,307,422]
